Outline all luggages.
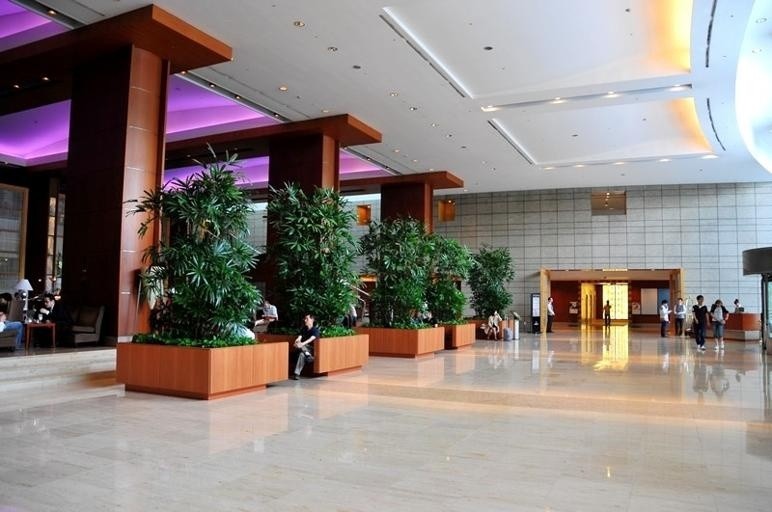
[503,317,513,341]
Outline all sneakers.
[304,357,314,363]
[696,344,725,350]
[294,374,300,380]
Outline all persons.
[660,337,670,374]
[601,300,611,326]
[710,300,729,349]
[692,295,710,350]
[673,298,686,335]
[659,300,672,337]
[261,300,279,333]
[675,336,685,376]
[0,292,74,351]
[547,333,555,369]
[293,313,319,380]
[547,297,555,333]
[486,309,502,340]
[693,348,709,401]
[605,326,610,351]
[487,340,502,370]
[734,299,742,307]
[710,349,729,398]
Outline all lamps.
[14,279,34,310]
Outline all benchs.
[444,326,456,349]
[257,336,318,375]
[469,320,507,340]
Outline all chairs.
[67,306,103,343]
[0,321,23,351]
